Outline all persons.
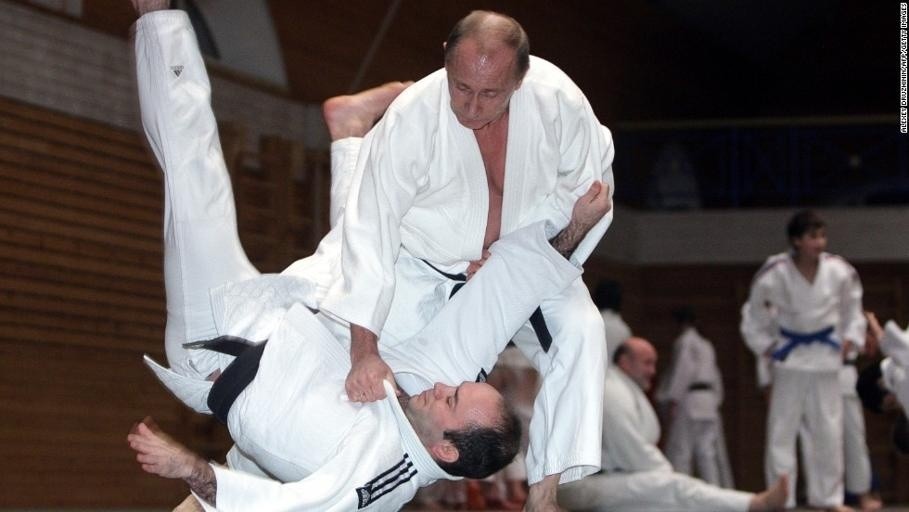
[126,0,613,512]
[317,8,614,512]
[407,208,909,511]
